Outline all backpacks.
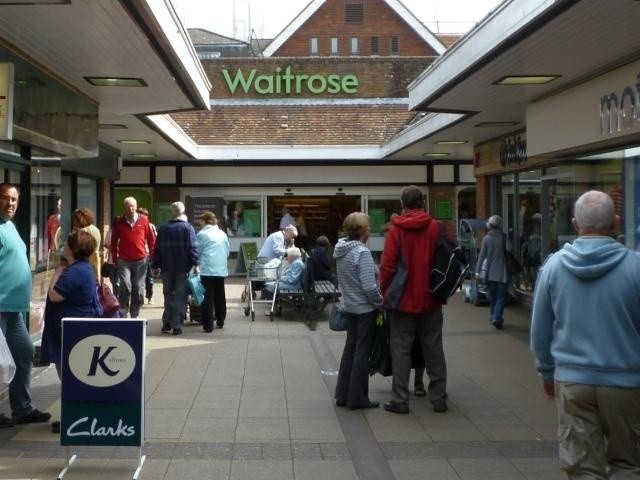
[430,222,470,301]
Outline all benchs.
[277,247,341,315]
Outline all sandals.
[415,381,425,398]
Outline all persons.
[529,190,639,480]
[1,184,52,426]
[475,215,513,329]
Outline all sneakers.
[161,322,171,331]
[10,407,54,422]
[0,411,15,430]
[336,400,380,409]
[432,401,448,413]
[173,328,182,335]
[384,400,408,416]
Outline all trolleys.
[241,263,282,322]
[185,273,216,327]
[458,217,489,305]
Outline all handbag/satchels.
[380,261,411,311]
[329,301,349,333]
[369,312,393,376]
[145,263,153,298]
[96,284,122,317]
[503,250,523,278]
